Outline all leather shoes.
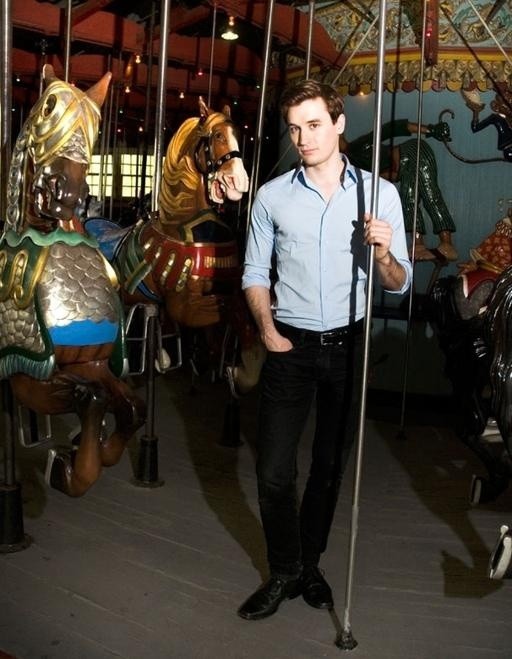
[299,566,334,608]
[238,570,305,620]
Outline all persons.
[235,79,414,619]
[466,98,512,158]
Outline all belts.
[273,316,362,347]
